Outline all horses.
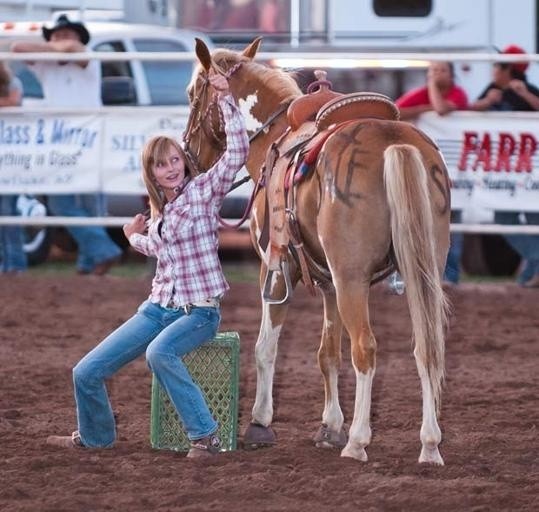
[181,36,451,467]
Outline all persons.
[11,15,124,276]
[0,60,27,274]
[46,66,250,458]
[468,45,539,288]
[395,62,468,289]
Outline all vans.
[0,22,219,251]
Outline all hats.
[42,15,90,46]
[492,39,530,74]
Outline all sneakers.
[186,432,220,458]
[76,258,119,276]
[46,430,84,449]
[517,258,539,286]
